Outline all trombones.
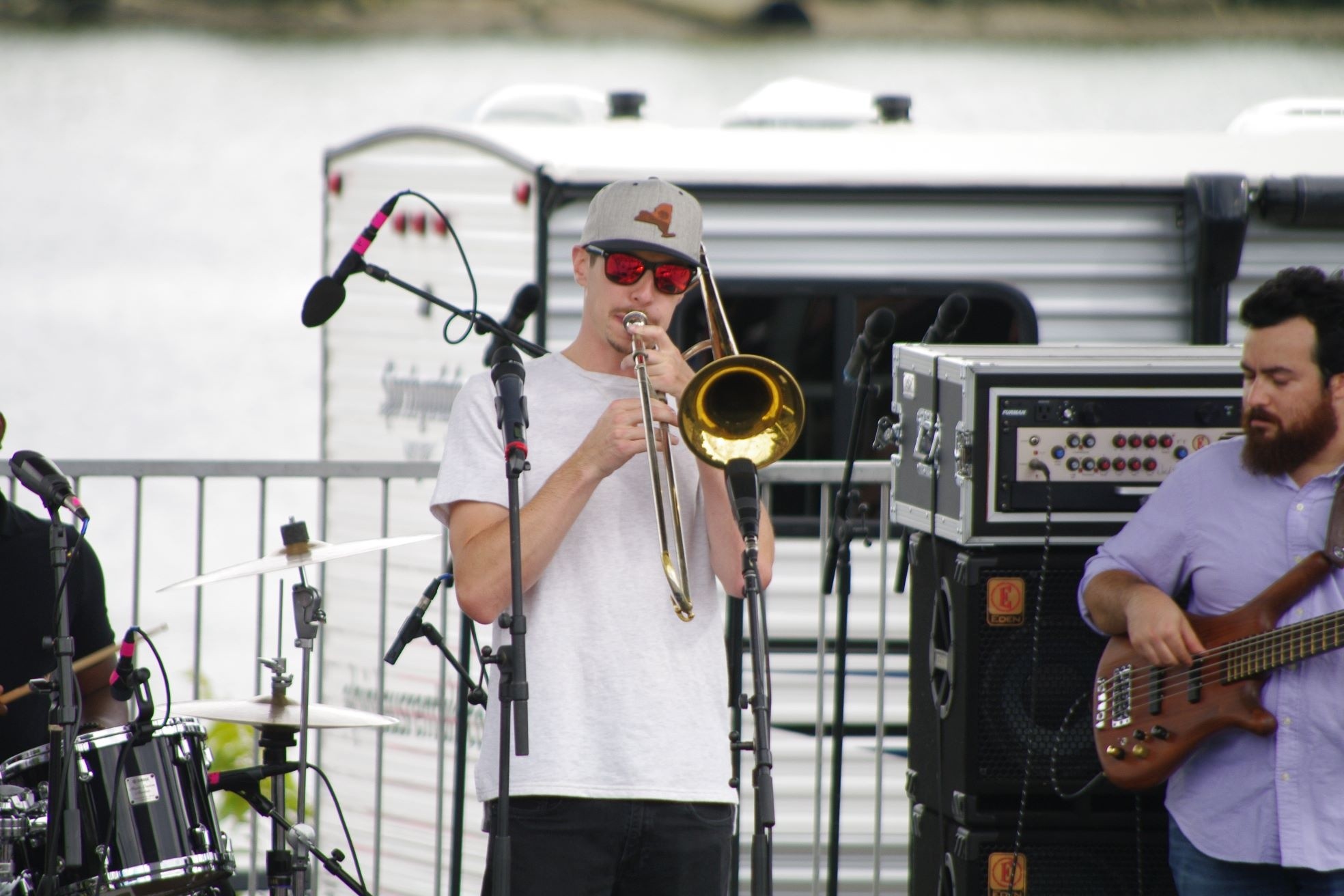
[622,243,806,624]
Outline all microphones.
[10,450,91,522]
[843,308,895,387]
[108,630,135,702]
[483,283,543,366]
[923,294,970,345]
[383,579,441,666]
[490,346,528,475]
[301,195,398,327]
[207,761,300,793]
[725,457,761,564]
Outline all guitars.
[1092,545,1344,785]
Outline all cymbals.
[155,698,401,728]
[152,530,443,594]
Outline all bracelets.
[78,722,107,736]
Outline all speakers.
[905,803,1179,896]
[907,531,1192,832]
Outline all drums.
[0,716,238,896]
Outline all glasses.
[587,245,698,294]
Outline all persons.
[1076,266,1344,896]
[429,176,776,896]
[0,414,130,896]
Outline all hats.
[580,176,709,274]
